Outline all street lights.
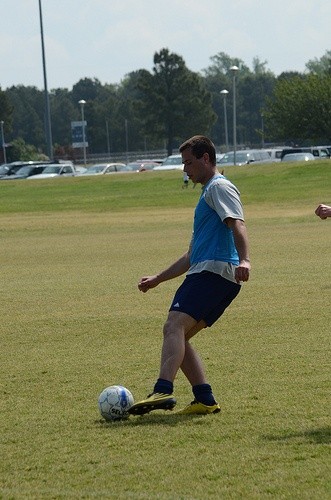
[220,89,228,153]
[0,120,7,163]
[228,65,239,165]
[78,99,87,167]
[261,113,264,148]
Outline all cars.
[77,162,135,175]
[127,144,331,173]
[0,158,87,180]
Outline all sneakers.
[131,392,177,417]
[173,398,221,415]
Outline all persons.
[127,136,252,415]
[315,204,331,219]
[183,172,204,190]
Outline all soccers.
[98,386,137,418]
